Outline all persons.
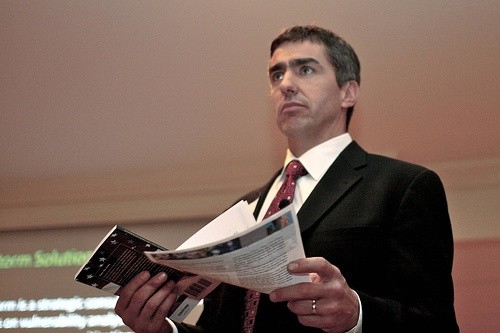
[115,25,460,333]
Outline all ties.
[240,158,308,333]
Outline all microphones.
[279,199,289,209]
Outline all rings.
[312,300,316,315]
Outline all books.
[74,226,222,324]
